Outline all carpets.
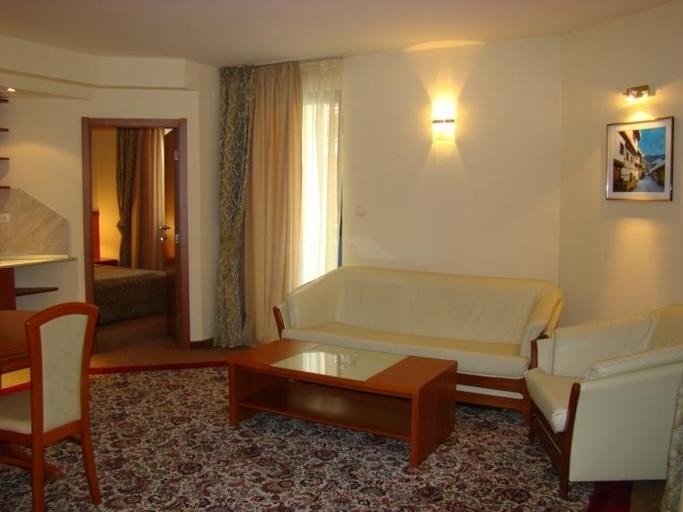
[0,358,590,511]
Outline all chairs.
[1,302,101,512]
[522,302,683,501]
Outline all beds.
[93,265,170,328]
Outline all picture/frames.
[604,117,674,200]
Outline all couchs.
[273,265,564,431]
